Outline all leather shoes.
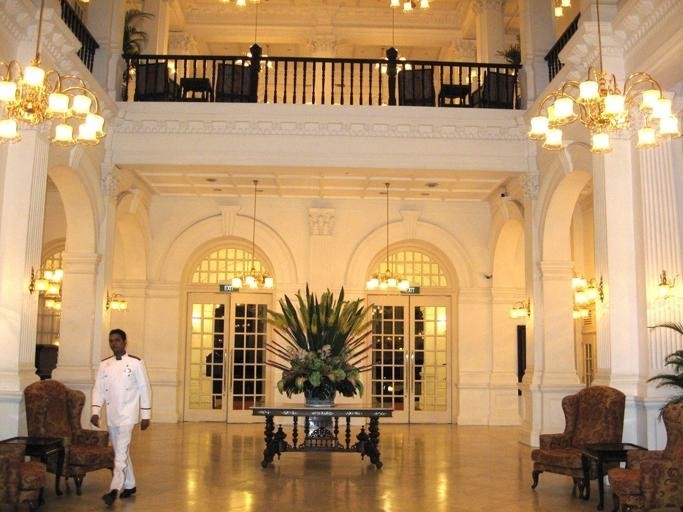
[119,486,137,499]
[103,489,117,505]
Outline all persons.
[91,330,152,506]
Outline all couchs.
[214,63,258,103]
[0,444,48,512]
[472,71,517,108]
[23,381,116,496]
[134,61,182,102]
[607,399,683,512]
[397,68,436,107]
[531,385,626,501]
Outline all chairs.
[39,292,62,310]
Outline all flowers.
[263,281,381,396]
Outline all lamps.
[657,269,682,302]
[0,0,106,148]
[366,182,410,292]
[572,274,603,321]
[374,13,413,75]
[231,180,275,290]
[236,0,274,73]
[105,292,129,312]
[216,0,265,9]
[389,0,434,14]
[510,298,531,320]
[30,266,63,298]
[553,0,572,17]
[527,0,682,154]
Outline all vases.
[299,382,343,448]
